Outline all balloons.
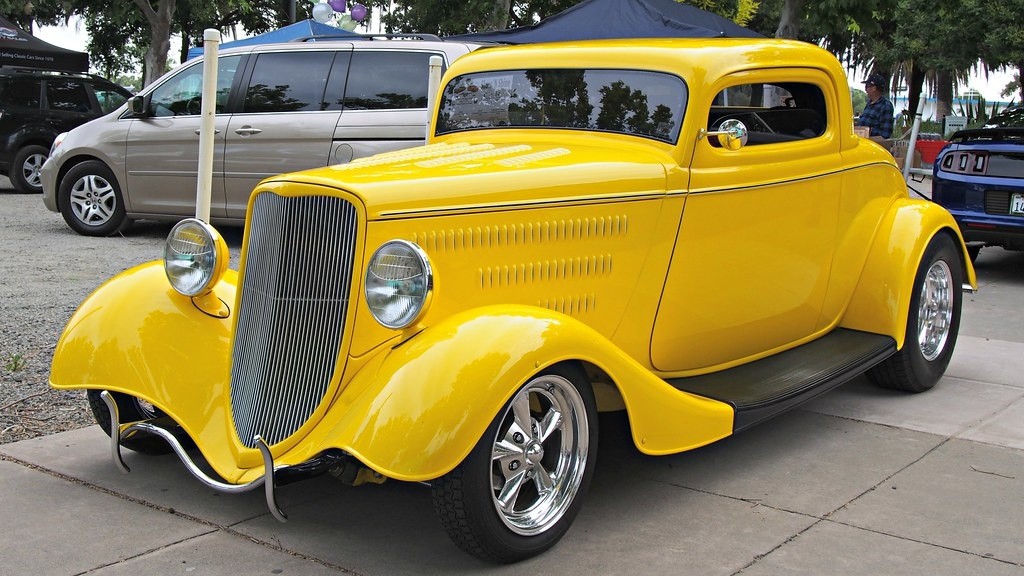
[312,0,367,31]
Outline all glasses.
[865,84,873,88]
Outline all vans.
[40,34,513,236]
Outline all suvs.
[0,66,135,194]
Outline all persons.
[857,73,894,139]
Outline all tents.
[187,19,381,61]
[0,16,90,74]
[441,0,770,44]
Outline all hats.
[861,73,885,91]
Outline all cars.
[932,104,1024,266]
[46,37,980,569]
